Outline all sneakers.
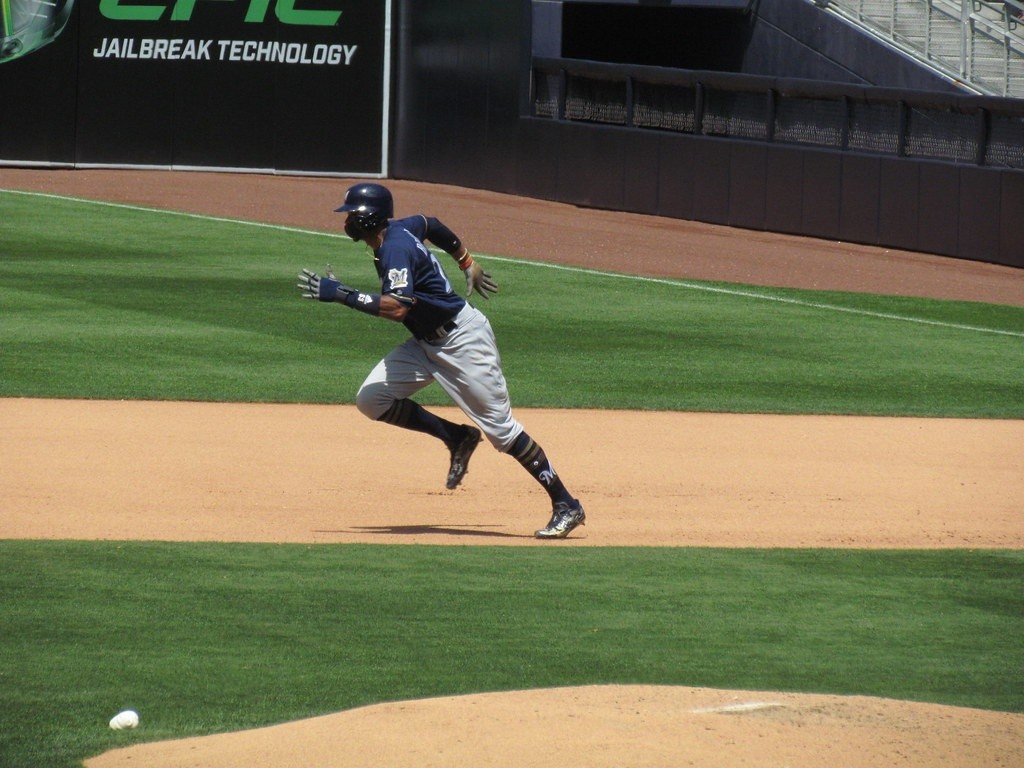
[446,424,481,489]
[535,499,585,538]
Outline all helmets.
[334,183,394,229]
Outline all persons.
[295,182,587,539]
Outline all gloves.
[457,248,498,299]
[297,264,356,304]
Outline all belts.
[423,303,474,342]
